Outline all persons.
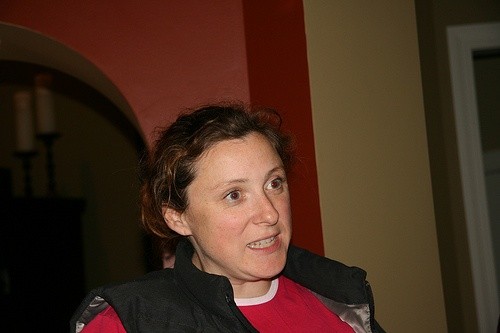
[70,102,389,333]
